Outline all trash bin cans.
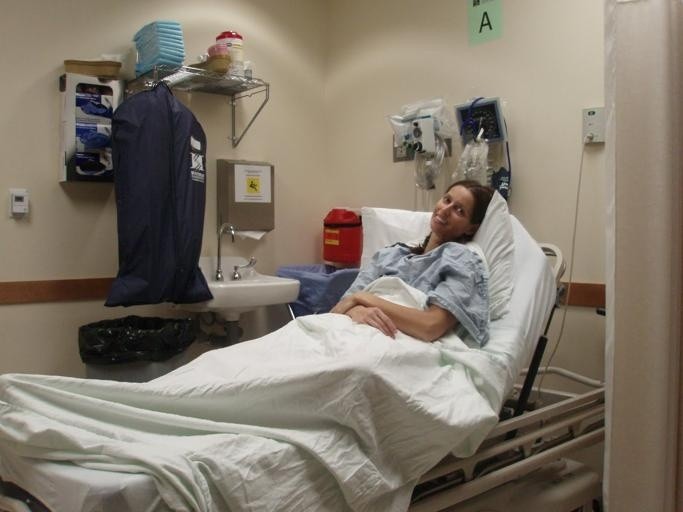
[78,316,192,380]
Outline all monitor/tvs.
[456,98,506,143]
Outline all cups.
[243,60,255,81]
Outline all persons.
[326,178,494,350]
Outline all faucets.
[217,224,235,270]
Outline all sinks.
[171,274,300,313]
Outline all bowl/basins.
[207,44,233,75]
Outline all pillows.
[360,190,513,321]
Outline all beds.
[0,212,606,512]
